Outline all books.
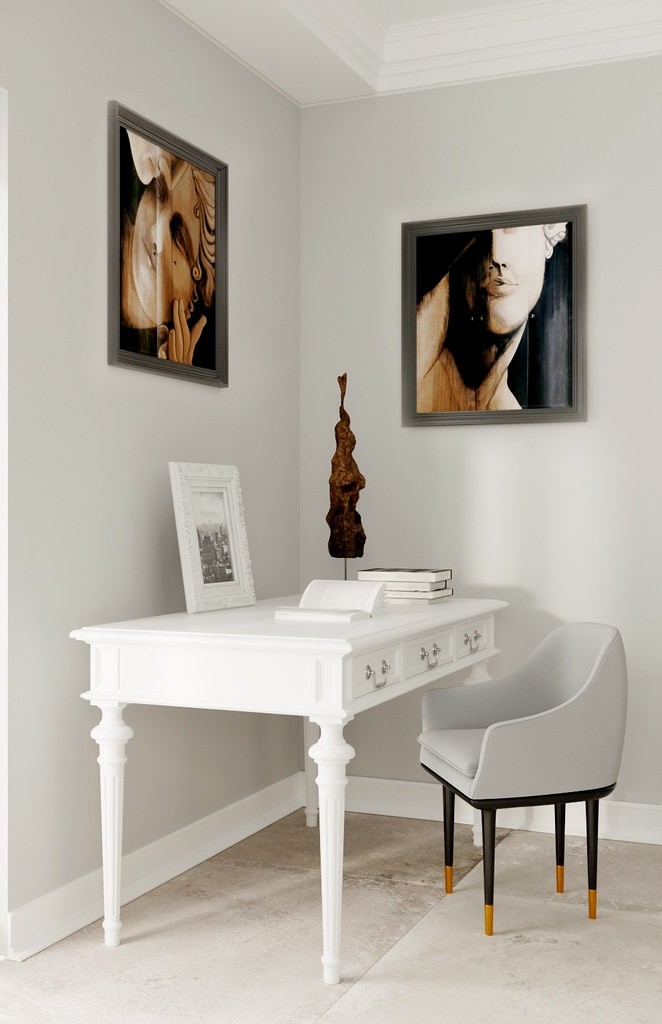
[273,579,390,623]
[356,566,454,605]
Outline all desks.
[69,598,513,985]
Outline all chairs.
[416,620,629,934]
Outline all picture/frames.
[401,203,588,425]
[106,98,229,390]
[169,462,257,611]
